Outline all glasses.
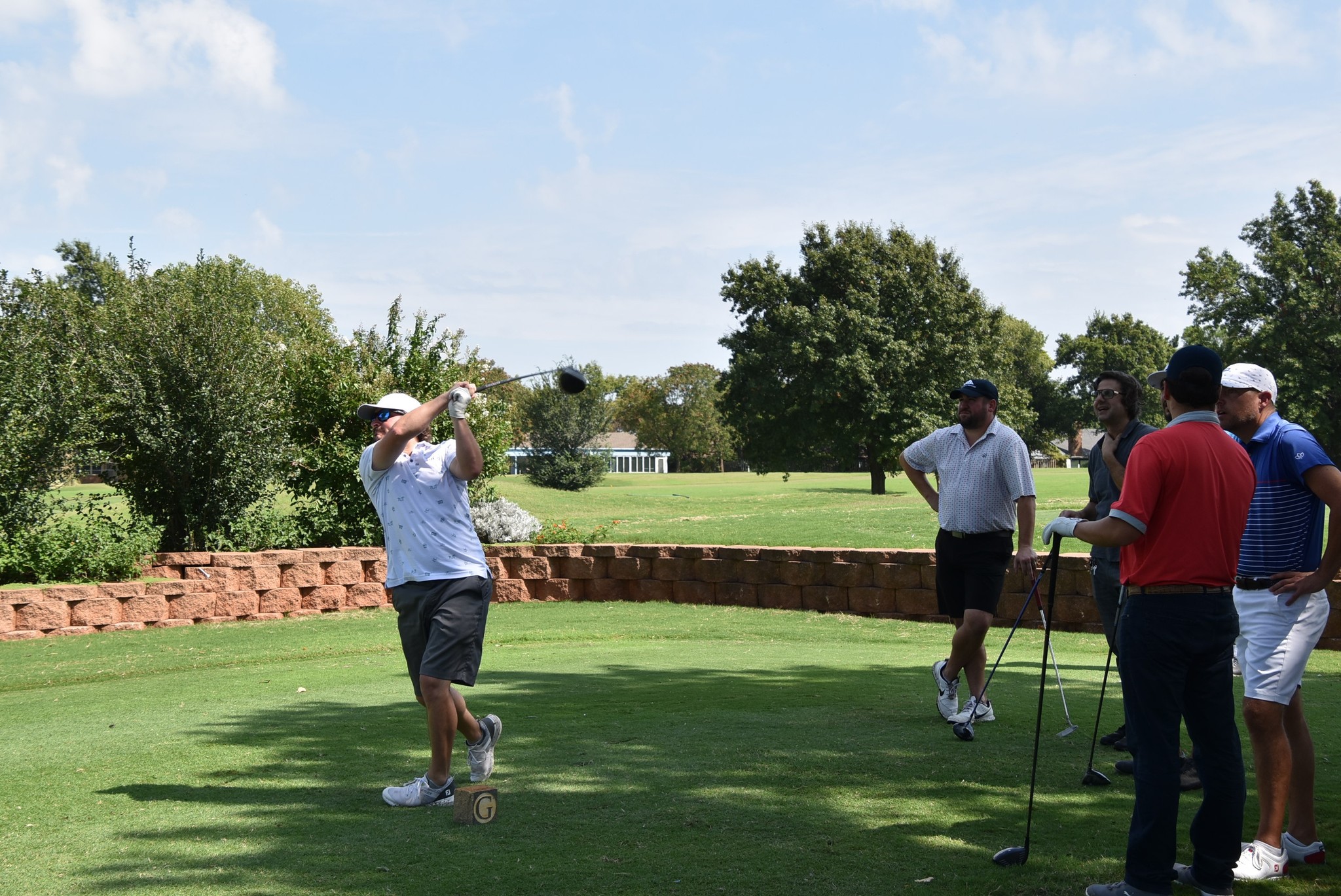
[1089,388,1126,401]
[376,409,405,422]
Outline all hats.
[1147,362,1169,389]
[1166,344,1223,407]
[1220,362,1278,405]
[357,393,419,421]
[950,379,1000,403]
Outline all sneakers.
[381,772,456,807]
[1172,862,1234,896]
[931,657,961,718]
[1281,832,1327,867]
[1233,832,1291,882]
[1085,879,1154,896]
[948,695,996,723]
[465,714,501,783]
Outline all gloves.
[1042,516,1088,546]
[448,387,472,420]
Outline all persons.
[1042,345,1254,896]
[1216,363,1340,882]
[899,379,1037,725]
[357,382,502,808]
[1058,362,1244,790]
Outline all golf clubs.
[952,526,1126,870]
[475,365,587,396]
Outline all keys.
[1087,557,1097,575]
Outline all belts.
[1232,575,1281,592]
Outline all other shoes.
[1100,723,1130,752]
[1179,752,1200,789]
[1114,759,1134,775]
